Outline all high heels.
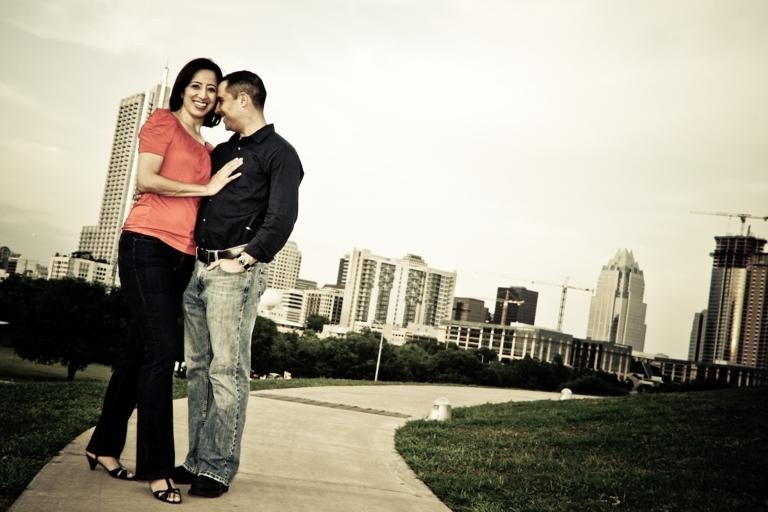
[86,451,133,479]
[152,478,182,504]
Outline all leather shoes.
[174,466,197,484]
[188,475,228,498]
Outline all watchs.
[236,252,250,271]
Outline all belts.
[196,245,249,264]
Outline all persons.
[134,70,305,498]
[85,57,246,504]
